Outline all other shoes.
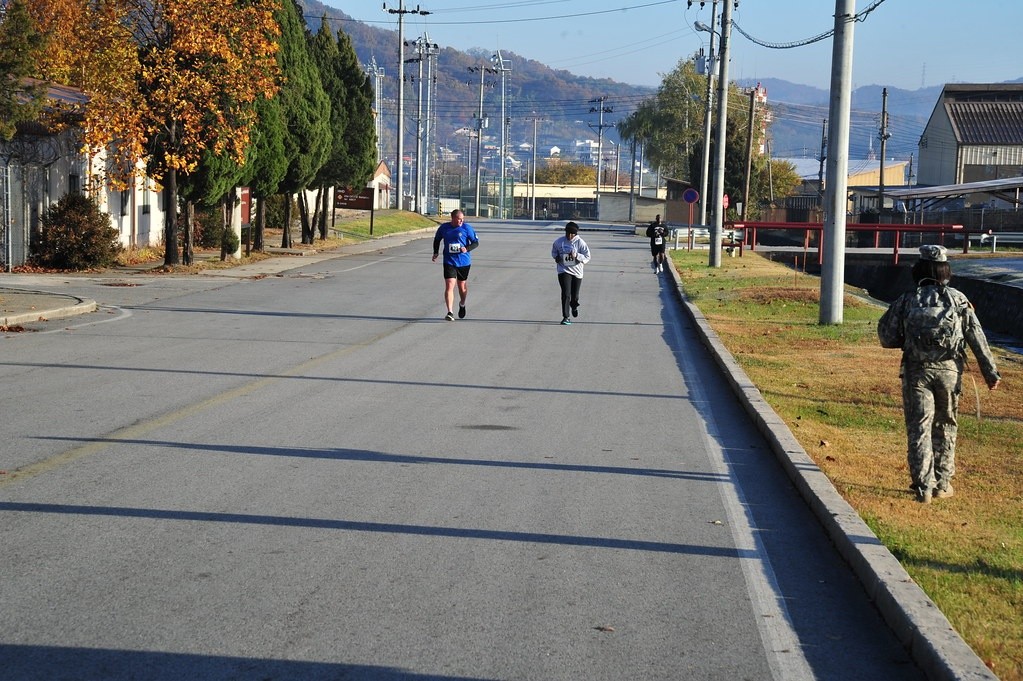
[572,307,578,317]
[561,318,572,325]
[919,492,932,504]
[933,484,953,498]
[445,313,454,322]
[458,301,466,319]
[658,262,664,273]
[655,266,659,274]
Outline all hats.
[920,244,947,264]
[564,222,578,233]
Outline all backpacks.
[904,277,964,361]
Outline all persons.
[432,209,479,321]
[552,222,590,325]
[646,214,670,274]
[877,244,1000,503]
